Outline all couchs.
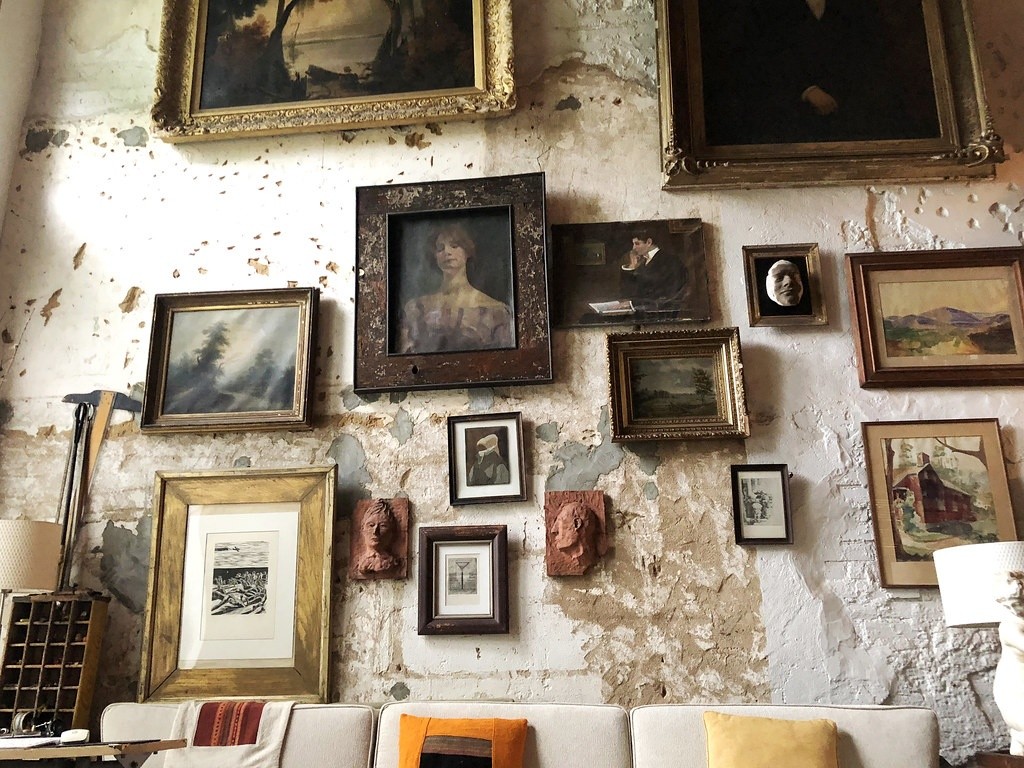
[99,702,941,768]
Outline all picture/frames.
[134,0,1024,702]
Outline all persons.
[992,570,1024,756]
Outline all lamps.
[0,520,64,633]
[933,541,1024,768]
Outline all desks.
[0,738,187,768]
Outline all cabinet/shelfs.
[0,593,111,738]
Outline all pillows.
[702,709,840,768]
[399,714,529,768]
